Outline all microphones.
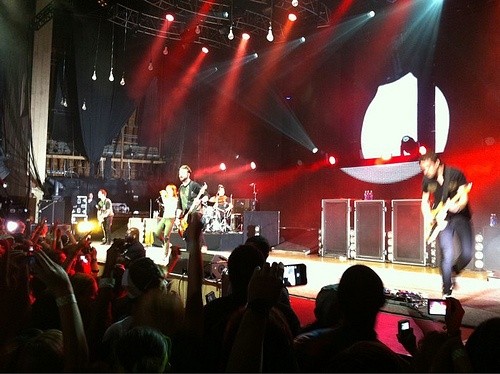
[155,197,160,202]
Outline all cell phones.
[280,264,307,287]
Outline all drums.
[205,201,232,219]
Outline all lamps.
[400,136,417,156]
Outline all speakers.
[320,199,441,267]
[167,199,281,281]
[483,226,500,271]
[129,218,160,245]
[39,200,69,225]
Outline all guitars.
[425,182,472,244]
[178,184,207,237]
[99,209,111,226]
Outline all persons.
[1,215,499,372]
[176,164,210,263]
[419,151,475,298]
[213,184,233,228]
[155,185,178,258]
[97,190,114,245]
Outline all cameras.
[428,298,447,316]
[398,320,410,335]
